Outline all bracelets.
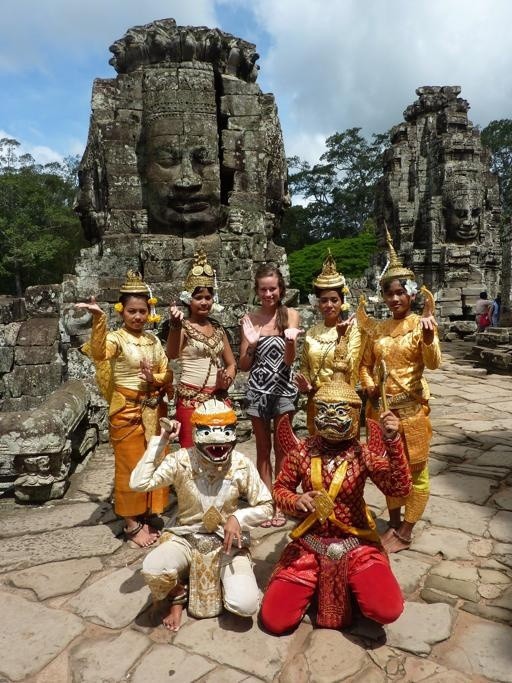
[244,345,256,358]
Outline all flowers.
[342,285,350,293]
[308,292,320,306]
[341,301,352,311]
[210,302,224,311]
[405,278,418,296]
[147,313,161,323]
[178,290,193,303]
[114,301,123,311]
[148,296,157,305]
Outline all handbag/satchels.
[480,314,490,327]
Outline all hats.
[184,248,214,293]
[379,220,415,287]
[120,271,149,294]
[314,255,345,289]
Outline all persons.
[359,266,444,556]
[292,256,360,449]
[261,381,413,637]
[489,292,501,326]
[447,191,482,240]
[144,106,225,230]
[130,401,262,633]
[472,290,492,331]
[167,249,237,449]
[239,268,303,529]
[74,272,171,549]
